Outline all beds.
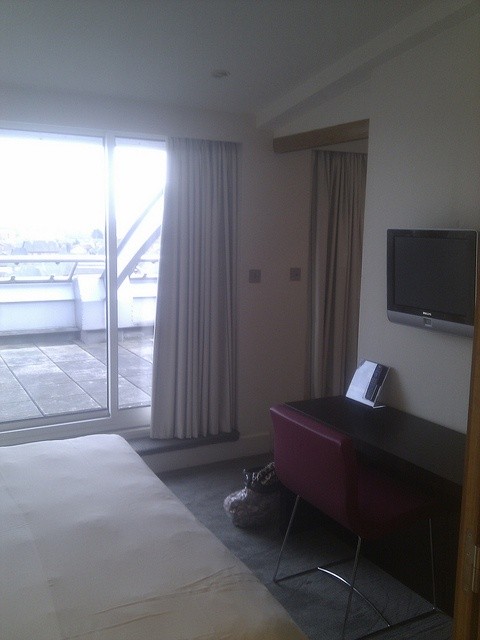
[0,434,309,640]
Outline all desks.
[279,395,467,539]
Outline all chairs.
[269,404,449,640]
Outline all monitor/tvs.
[387,229,477,339]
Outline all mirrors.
[345,359,391,407]
